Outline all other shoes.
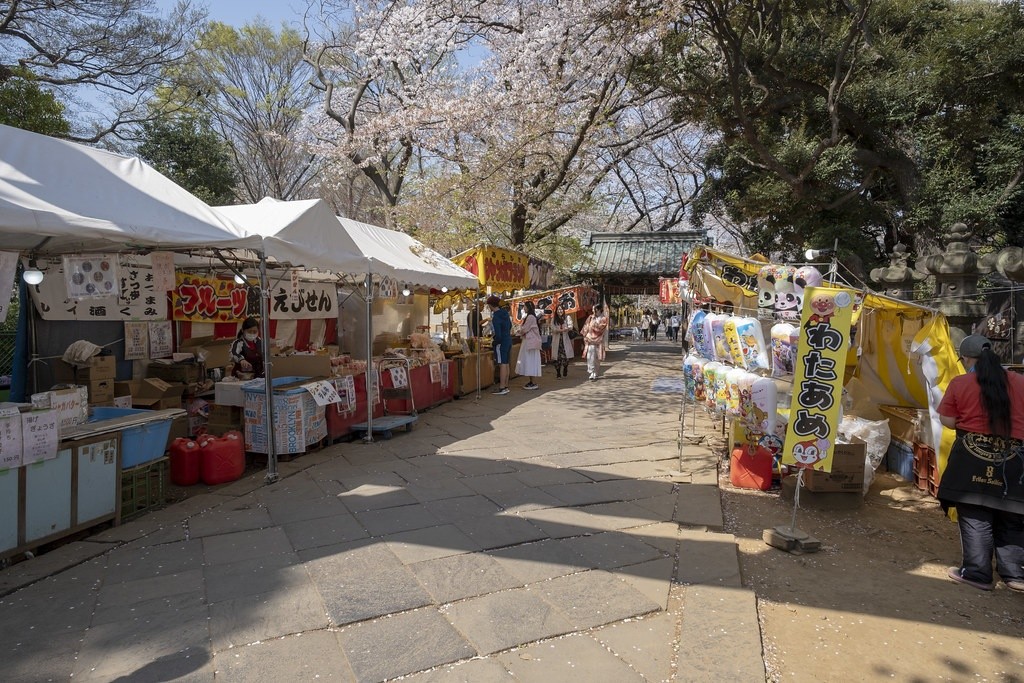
[589,372,596,380]
[557,370,562,379]
[995,566,1024,591]
[947,567,995,591]
[563,368,568,378]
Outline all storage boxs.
[54,336,244,451]
[323,346,339,356]
[121,460,171,522]
[729,420,785,470]
[802,432,867,493]
[878,405,940,498]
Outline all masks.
[243,330,258,341]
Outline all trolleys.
[351,358,420,440]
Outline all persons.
[486,296,513,395]
[641,308,680,342]
[467,300,491,337]
[580,303,607,380]
[229,316,262,379]
[515,301,541,390]
[936,335,1024,594]
[551,304,574,379]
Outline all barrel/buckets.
[730,442,772,490]
[170,430,244,484]
[729,419,758,455]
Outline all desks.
[325,342,524,449]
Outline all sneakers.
[492,387,510,395]
[523,381,538,389]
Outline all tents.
[0,124,480,483]
[428,242,600,346]
[678,241,969,522]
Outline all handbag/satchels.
[565,314,578,340]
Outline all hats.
[487,296,500,304]
[957,334,992,362]
